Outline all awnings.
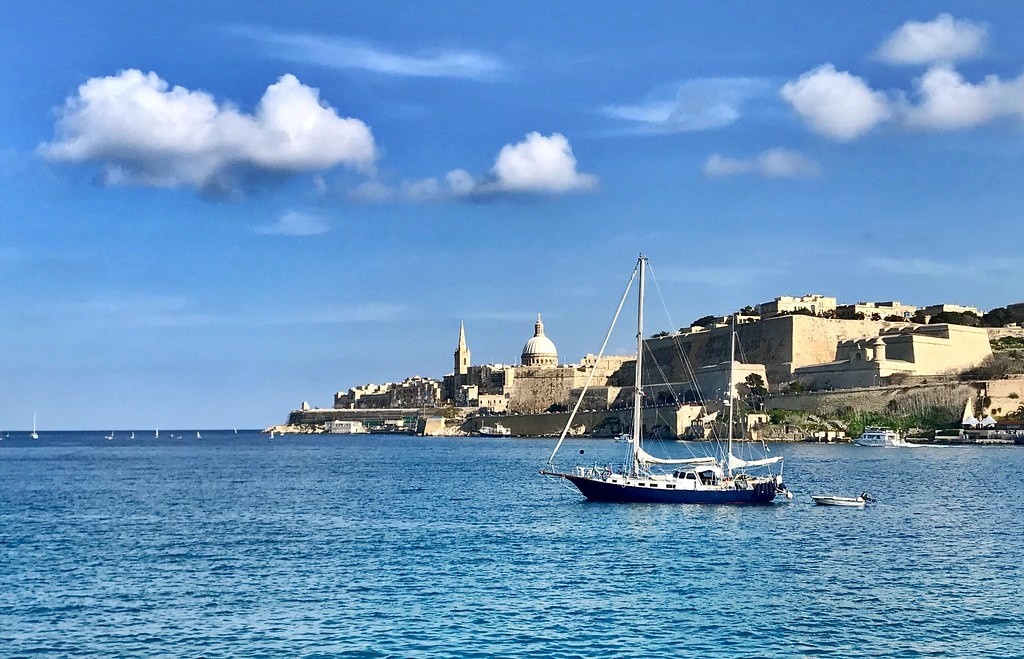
[727,451,784,470]
[638,447,716,464]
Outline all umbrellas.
[962,414,998,428]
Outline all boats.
[811,493,867,507]
[853,429,902,447]
[479,422,510,437]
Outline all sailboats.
[30,411,39,438]
[539,253,793,504]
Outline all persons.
[775,473,783,490]
